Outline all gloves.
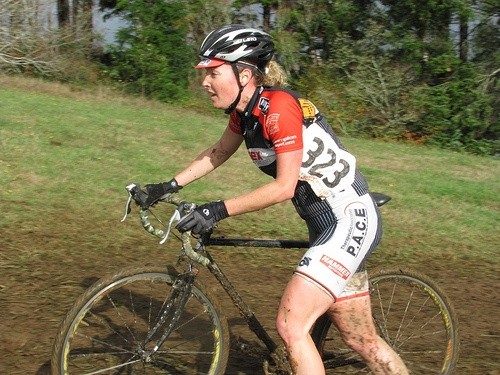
[138,177,182,211]
[174,198,229,236]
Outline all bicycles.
[50,185,461,375]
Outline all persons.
[130,24,410,375]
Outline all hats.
[194,58,226,68]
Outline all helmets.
[193,25,276,75]
